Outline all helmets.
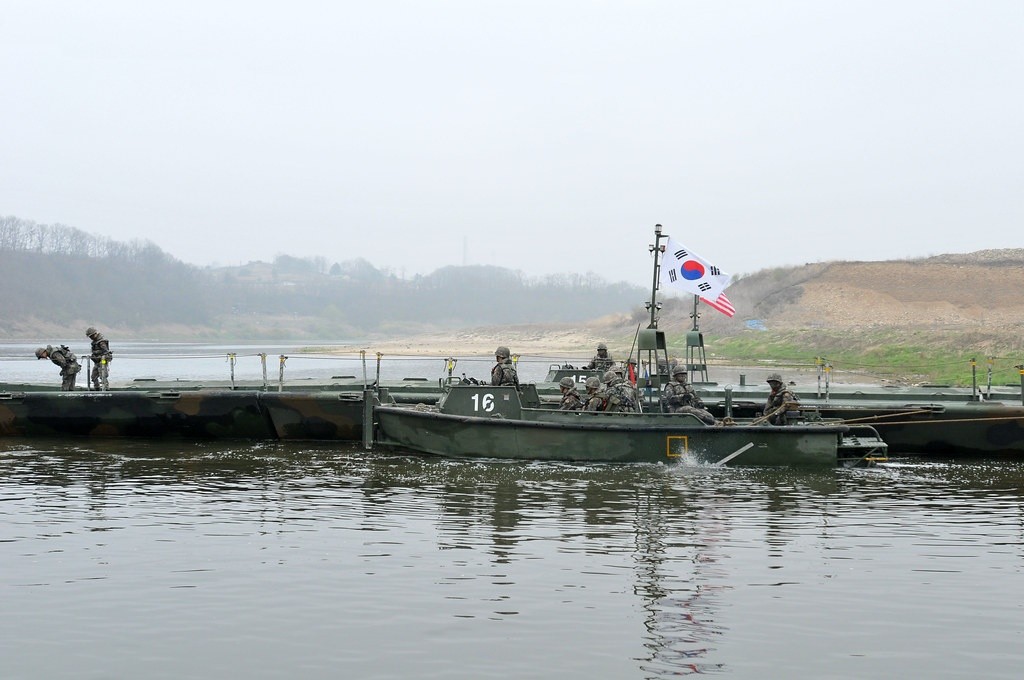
[766,373,783,385]
[672,365,688,377]
[584,377,601,388]
[85,327,98,338]
[495,346,510,358]
[609,365,622,373]
[35,347,46,360]
[602,371,617,384]
[558,377,574,389]
[597,343,607,351]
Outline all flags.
[699,292,736,318]
[659,236,729,303]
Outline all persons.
[489,347,521,397]
[35,345,81,391]
[661,365,722,426]
[763,374,801,426]
[85,327,109,391]
[551,343,678,417]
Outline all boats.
[372,223,849,468]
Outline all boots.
[702,415,723,425]
